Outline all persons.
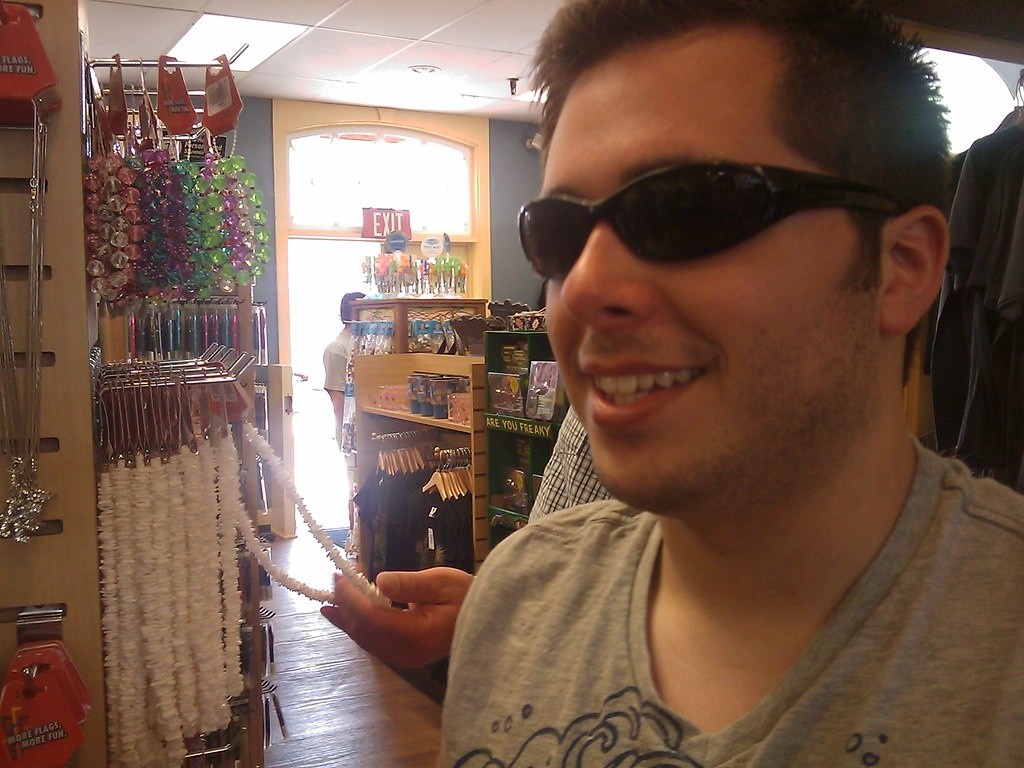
[323,292,364,531]
[436,0,1024,768]
[321,406,615,666]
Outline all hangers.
[375,428,432,477]
[422,450,473,502]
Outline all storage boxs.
[500,436,544,518]
[486,359,568,423]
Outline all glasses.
[517,158,908,281]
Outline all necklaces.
[1,95,54,541]
[97,365,392,768]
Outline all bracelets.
[84,151,269,314]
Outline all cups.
[374,371,471,427]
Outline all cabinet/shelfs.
[344,301,490,575]
[485,329,564,555]
